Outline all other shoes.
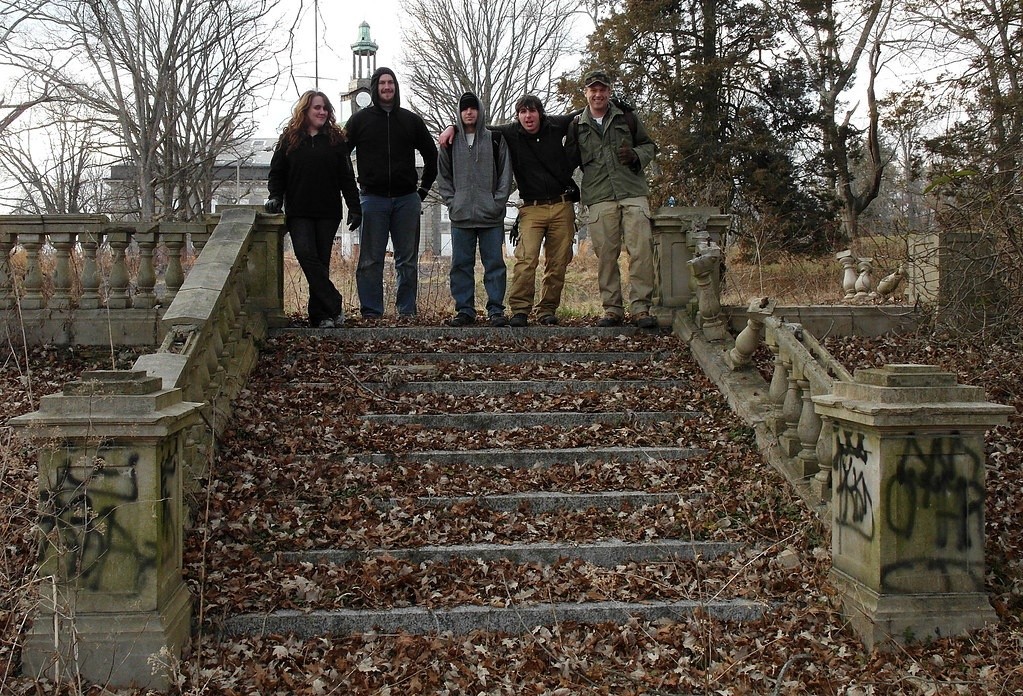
[355,314,382,327]
[398,315,420,327]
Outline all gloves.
[417,188,428,202]
[265,196,283,214]
[612,98,634,113]
[347,212,362,232]
[617,142,638,165]
[509,219,519,246]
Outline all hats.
[584,70,609,87]
[459,93,479,112]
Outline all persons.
[265,67,438,329]
[437,70,660,327]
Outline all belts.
[516,196,573,207]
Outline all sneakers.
[336,309,345,326]
[538,315,559,326]
[630,312,654,326]
[596,312,623,327]
[446,312,473,327]
[511,313,529,327]
[489,314,507,326]
[319,318,334,328]
